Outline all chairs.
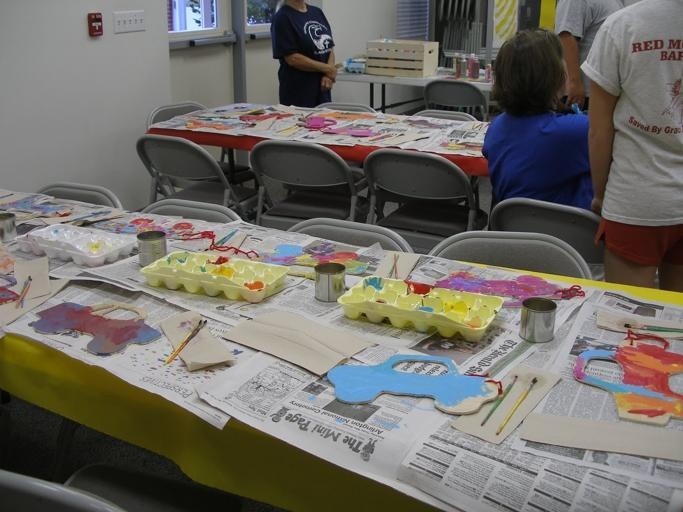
[249,141,369,229]
[37,181,123,210]
[136,135,268,221]
[149,99,206,126]
[287,217,415,252]
[429,230,592,280]
[487,198,602,280]
[314,78,490,122]
[142,199,242,222]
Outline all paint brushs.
[481,375,518,427]
[166,319,208,364]
[495,377,537,436]
[16,275,31,308]
[214,229,249,251]
[389,253,401,279]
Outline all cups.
[518,297,558,343]
[313,262,346,302]
[135,230,167,268]
[0,212,17,243]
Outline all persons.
[481,27,593,210]
[269,0,337,106]
[581,0,683,292]
[553,0,624,112]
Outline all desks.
[146,102,490,185]
[0,188,681,512]
[335,67,493,122]
